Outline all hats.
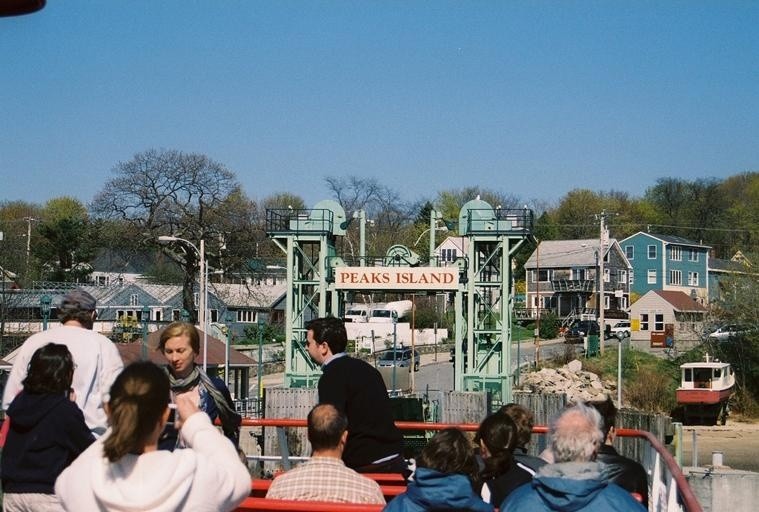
[61,288,97,311]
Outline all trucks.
[344,303,386,324]
[370,299,418,324]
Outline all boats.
[675,353,737,406]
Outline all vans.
[702,322,758,344]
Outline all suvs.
[562,321,612,344]
[613,321,632,341]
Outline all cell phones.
[164,403,178,425]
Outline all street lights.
[265,265,287,272]
[39,293,51,331]
[155,234,208,381]
[615,328,625,410]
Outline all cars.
[379,348,423,374]
[449,337,467,359]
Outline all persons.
[54,359,252,512]
[1,290,124,443]
[1,344,96,512]
[262,404,386,506]
[304,316,403,472]
[385,397,648,512]
[157,322,241,453]
